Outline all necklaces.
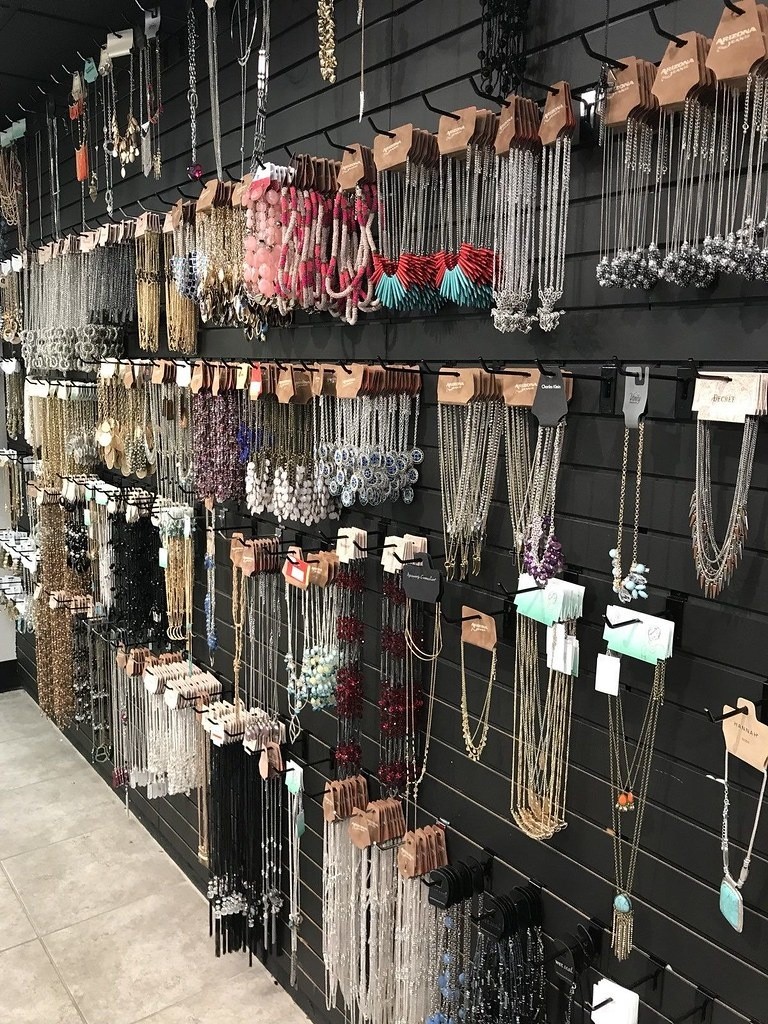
[0,0,768,1023]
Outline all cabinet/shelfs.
[0,0,768,1024]
